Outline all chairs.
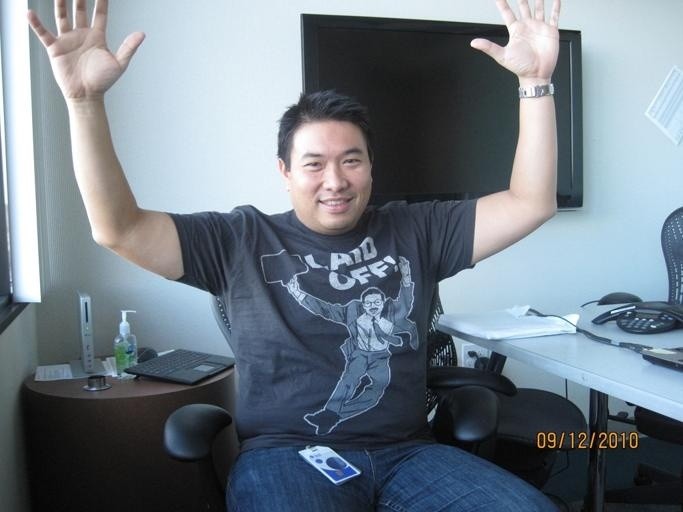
[426,294,588,492]
[597,207,683,487]
[164,291,501,503]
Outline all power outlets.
[462,343,491,368]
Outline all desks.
[434,303,683,512]
[22,355,239,512]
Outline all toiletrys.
[114,310,138,380]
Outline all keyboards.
[124,349,235,386]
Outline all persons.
[26,0,563,511]
[287,255,418,437]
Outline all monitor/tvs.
[300,13,583,212]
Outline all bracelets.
[517,82,554,98]
